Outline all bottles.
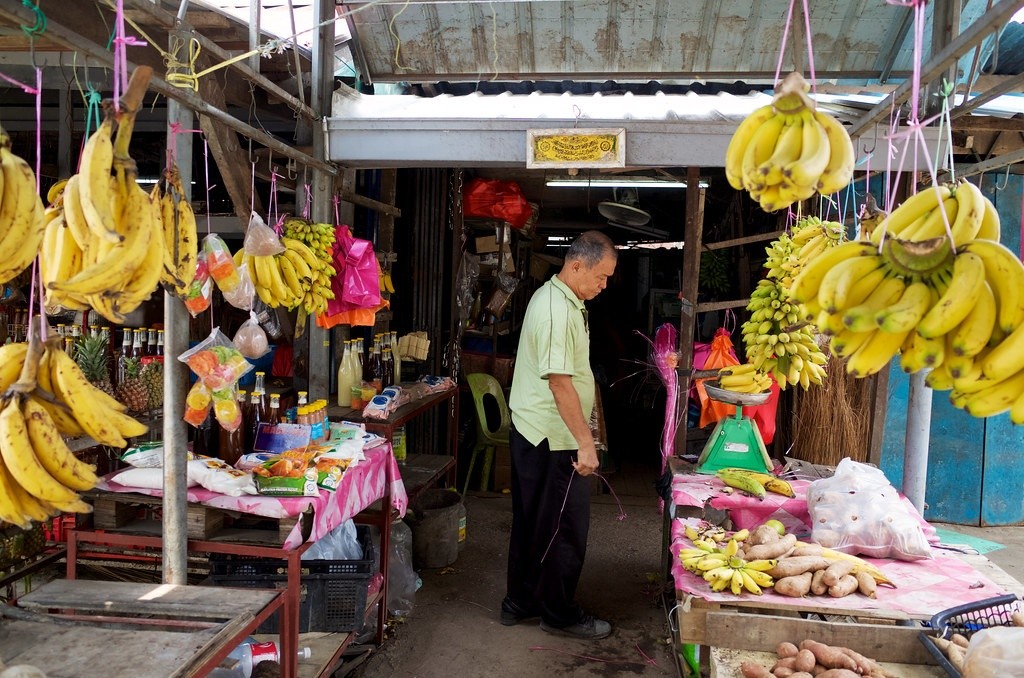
[54,325,165,393]
[226,641,312,678]
[337,332,402,409]
[192,371,330,467]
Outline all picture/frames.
[526,128,626,168]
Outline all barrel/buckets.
[406,488,463,568]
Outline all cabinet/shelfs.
[464,219,532,352]
[67,443,410,678]
[327,382,459,622]
[660,455,1024,678]
[0,417,163,588]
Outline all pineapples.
[75,329,163,410]
[1,523,46,567]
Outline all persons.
[500,229,618,640]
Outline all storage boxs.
[475,235,499,253]
[461,350,515,388]
[207,525,375,634]
[490,446,511,490]
[40,512,92,543]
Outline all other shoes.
[500,595,542,626]
[540,614,611,639]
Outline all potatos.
[741,639,901,678]
[808,468,932,562]
[736,524,878,597]
[928,611,1024,678]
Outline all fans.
[598,187,651,225]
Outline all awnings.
[337,0,1013,83]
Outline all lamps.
[544,174,712,189]
[607,220,669,241]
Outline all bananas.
[678,525,779,595]
[379,267,395,293]
[726,93,854,212]
[232,222,339,334]
[0,315,149,531]
[0,65,198,323]
[719,177,1024,424]
[716,468,797,500]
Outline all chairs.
[463,373,511,494]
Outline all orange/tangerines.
[765,520,784,535]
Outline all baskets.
[917,593,1024,678]
[213,523,373,633]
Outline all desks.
[0,580,290,678]
